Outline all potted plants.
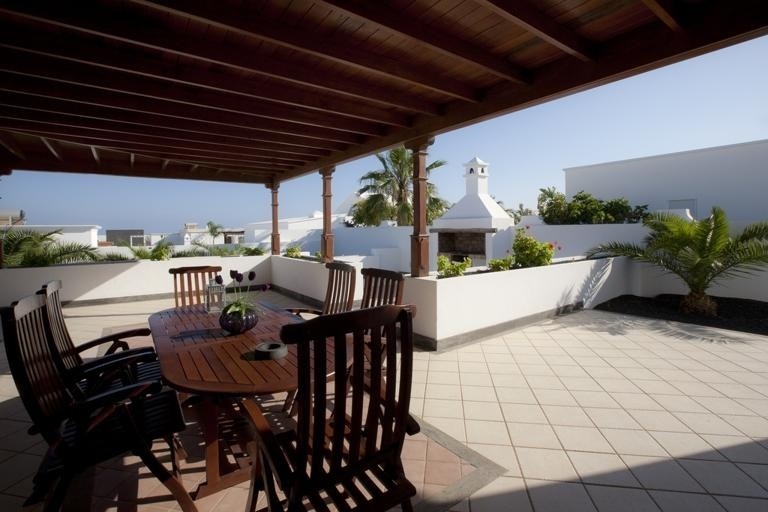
[213,269,258,333]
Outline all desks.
[148,297,354,500]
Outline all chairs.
[168,266,222,307]
[243,301,418,512]
[282,260,405,423]
[3,280,198,512]
